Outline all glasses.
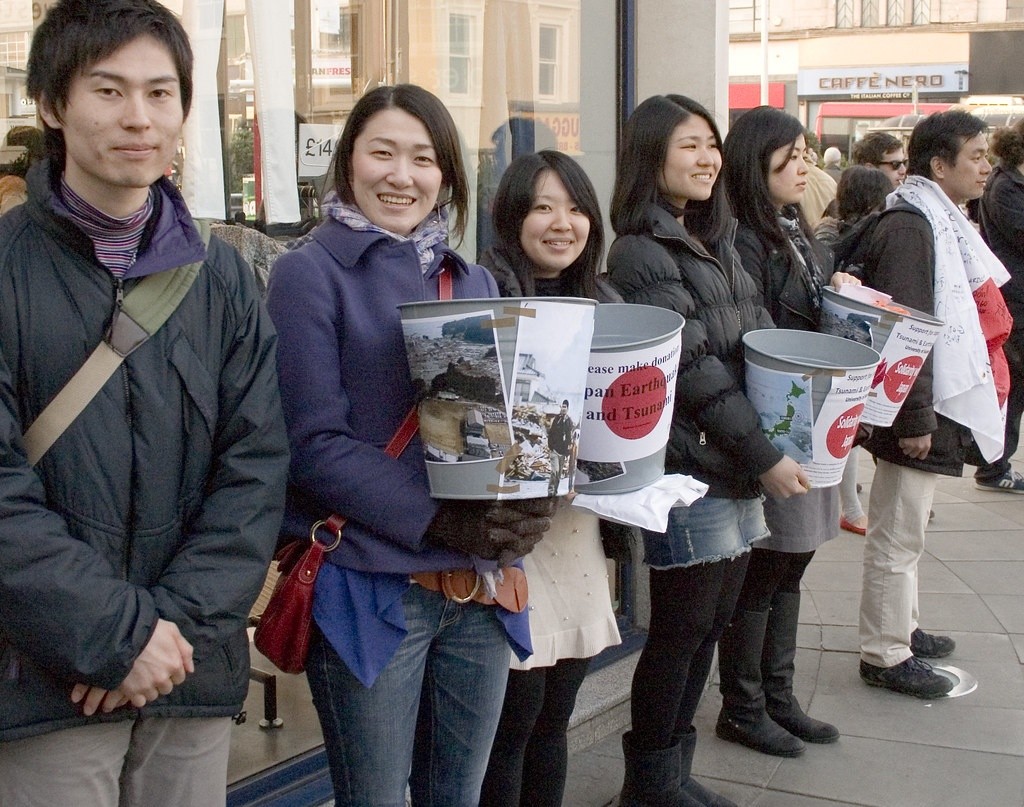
[875,157,908,170]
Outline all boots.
[716,606,807,755]
[620,730,707,807]
[761,592,840,741]
[672,725,738,807]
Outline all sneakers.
[910,628,955,657]
[859,656,954,696]
[974,467,1024,494]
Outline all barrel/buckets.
[395,296,600,501]
[819,285,946,428]
[742,329,883,490]
[573,303,686,495]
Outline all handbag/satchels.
[253,535,326,674]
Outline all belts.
[409,565,528,613]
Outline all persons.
[799,128,909,230]
[267,83,556,807]
[0,0,290,807]
[859,109,1015,700]
[0,126,43,214]
[813,165,895,535]
[548,399,574,496]
[715,106,862,757]
[974,113,1024,495]
[607,92,811,807]
[477,150,626,807]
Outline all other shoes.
[839,515,867,534]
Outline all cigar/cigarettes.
[899,180,903,185]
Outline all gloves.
[426,497,559,567]
